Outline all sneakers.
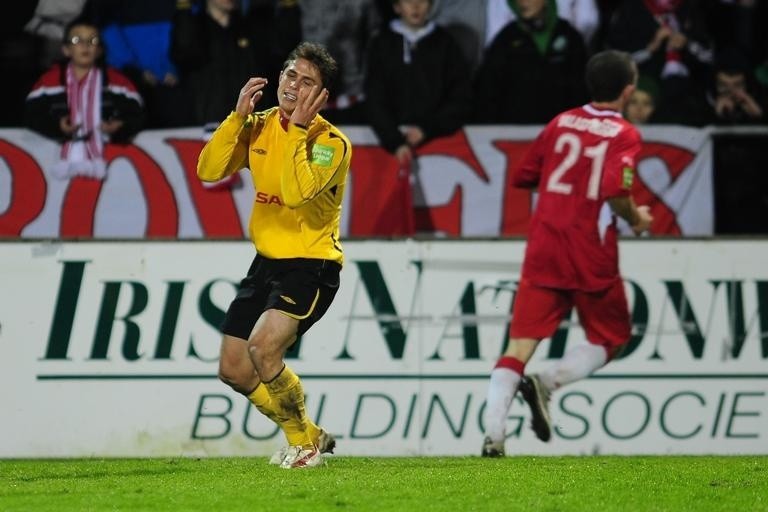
[278,444,323,469]
[480,434,507,457]
[520,373,554,442]
[268,428,334,466]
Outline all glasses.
[63,33,100,47]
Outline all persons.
[483,48,655,458]
[2,1,765,161]
[199,37,354,469]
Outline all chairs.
[105,24,185,83]
